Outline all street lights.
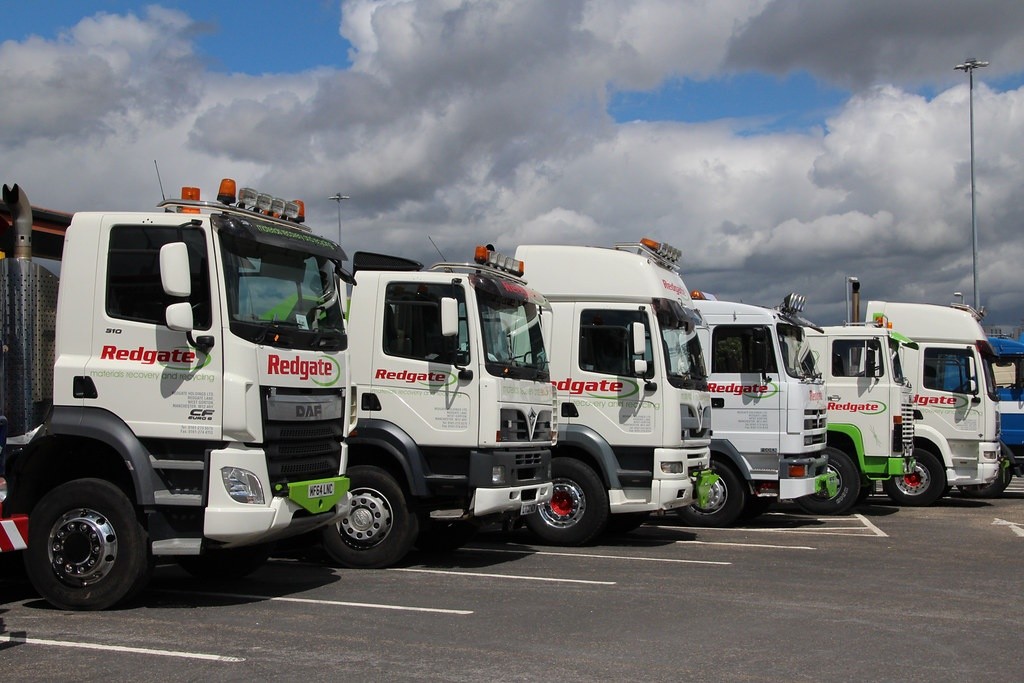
[326,192,350,251]
[953,58,989,324]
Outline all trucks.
[1,176,358,613]
[455,239,719,547]
[646,273,1024,529]
[241,243,560,571]
